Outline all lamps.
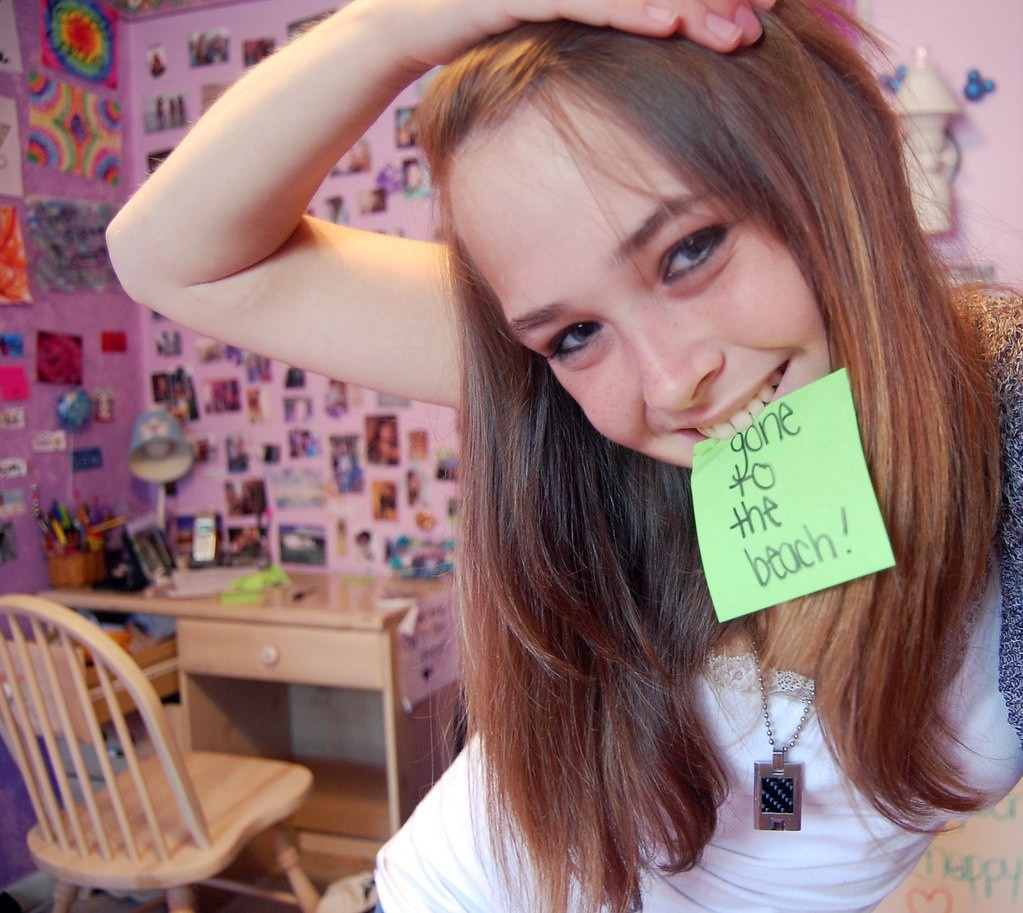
[128,407,196,532]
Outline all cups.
[49,551,105,587]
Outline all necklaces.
[746,618,817,832]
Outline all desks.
[23,569,458,884]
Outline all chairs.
[0,593,323,913]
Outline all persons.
[104,0,1023,913]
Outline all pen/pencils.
[36,492,107,552]
